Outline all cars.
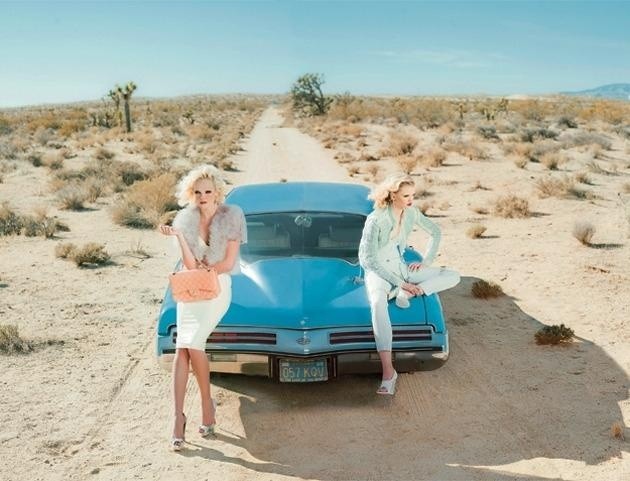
[151,176,456,380]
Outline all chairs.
[239,226,291,250]
[317,223,364,249]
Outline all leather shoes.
[198,396,219,437]
[169,413,187,451]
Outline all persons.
[358,172,462,396]
[158,164,248,451]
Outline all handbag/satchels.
[167,256,223,303]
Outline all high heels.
[388,286,410,310]
[374,367,399,396]
